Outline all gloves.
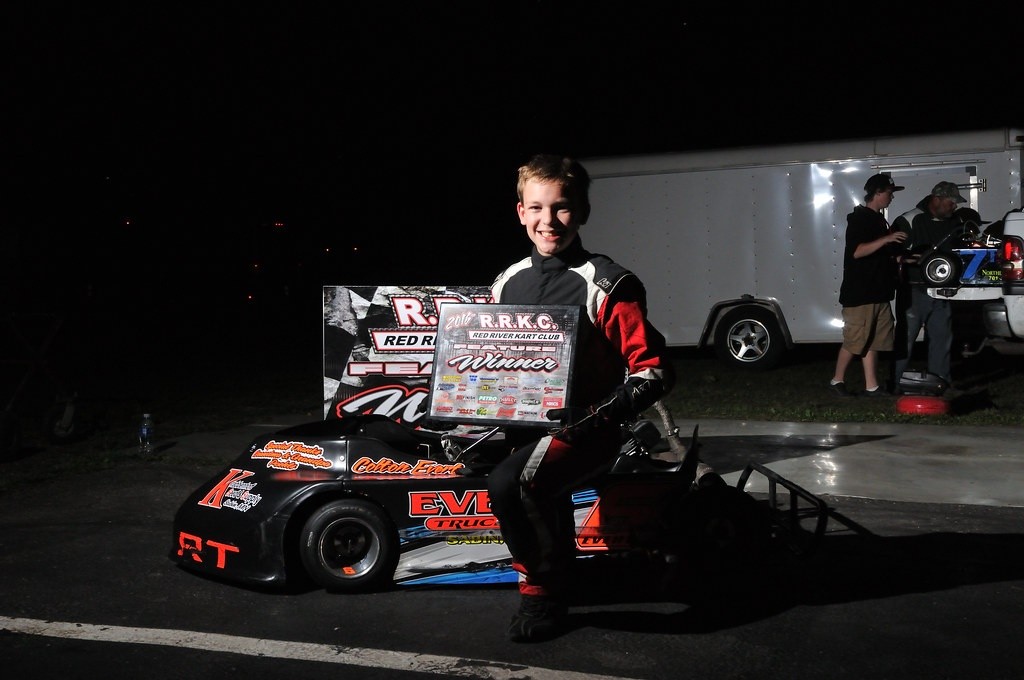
[546,388,630,447]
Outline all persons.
[494,155,674,641]
[819,174,908,400]
[888,182,967,399]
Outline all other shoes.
[506,586,570,640]
[943,387,967,401]
[864,385,893,397]
[827,380,851,398]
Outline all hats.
[864,174,905,192]
[933,181,967,203]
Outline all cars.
[980,206,1024,359]
